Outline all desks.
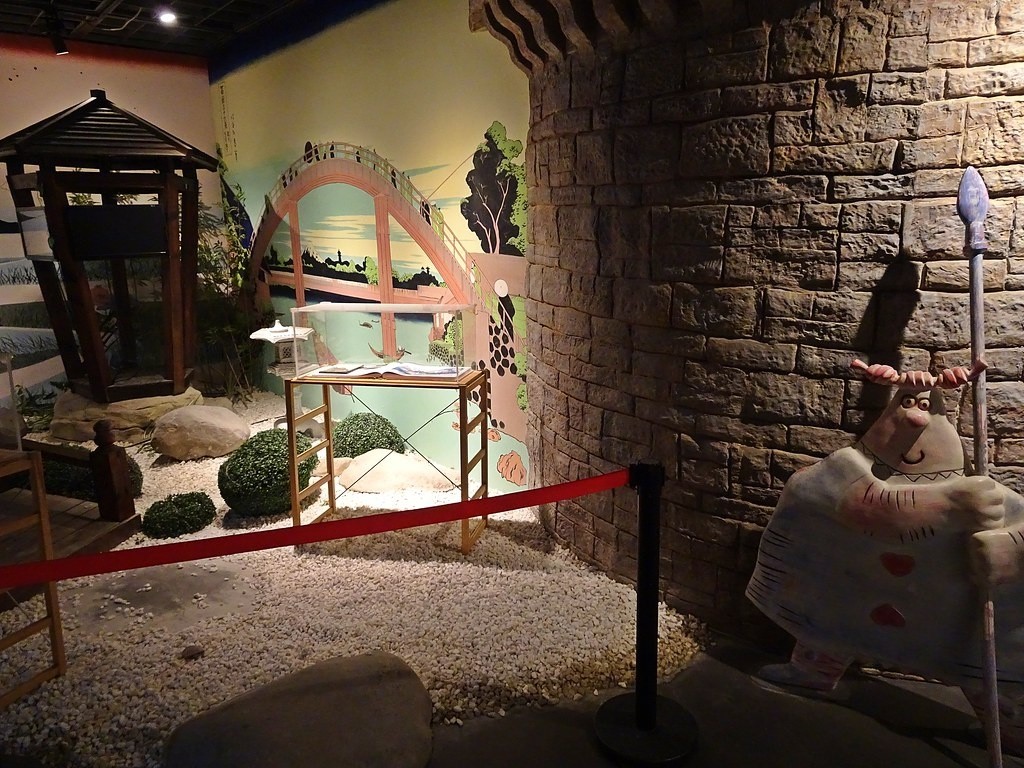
[285,365,490,555]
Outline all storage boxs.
[290,301,473,382]
